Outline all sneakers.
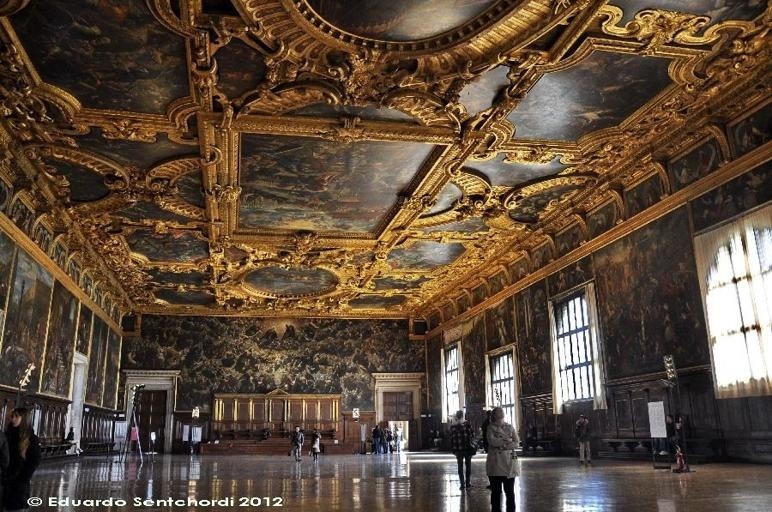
[461,483,471,488]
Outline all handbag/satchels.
[467,438,478,455]
[308,449,313,457]
[288,450,292,456]
[506,451,521,479]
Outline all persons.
[653,415,675,456]
[450,405,520,512]
[373,421,391,454]
[66,426,84,455]
[129,422,138,453]
[0,398,41,512]
[310,428,322,461]
[575,413,592,463]
[291,425,305,462]
